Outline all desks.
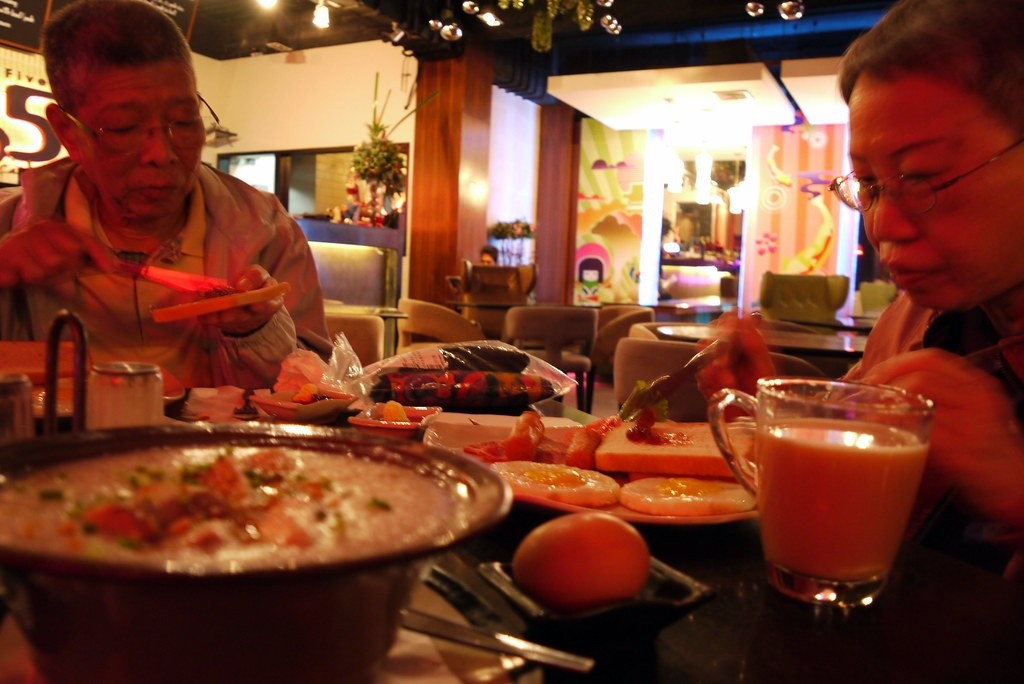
[656,325,871,359]
[444,297,601,310]
[601,295,738,323]
[834,316,876,331]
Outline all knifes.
[109,259,229,293]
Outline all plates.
[424,420,758,528]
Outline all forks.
[619,310,764,422]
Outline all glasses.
[824,135,1024,222]
[58,89,222,153]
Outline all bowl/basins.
[249,389,360,422]
[347,405,443,438]
[0,420,514,684]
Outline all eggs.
[489,459,622,508]
[618,475,756,516]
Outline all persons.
[480,244,498,265]
[0,0,334,386]
[695,0,1024,525]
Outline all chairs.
[305,239,897,424]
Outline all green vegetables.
[19,445,392,551]
[619,380,670,423]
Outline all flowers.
[493,219,536,240]
[352,68,441,196]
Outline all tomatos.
[510,511,651,613]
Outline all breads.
[593,421,751,474]
[152,282,290,323]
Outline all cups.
[706,374,937,611]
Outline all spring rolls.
[505,411,547,461]
[564,427,603,471]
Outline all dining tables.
[167,397,1024,684]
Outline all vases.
[361,177,387,227]
[497,239,523,262]
